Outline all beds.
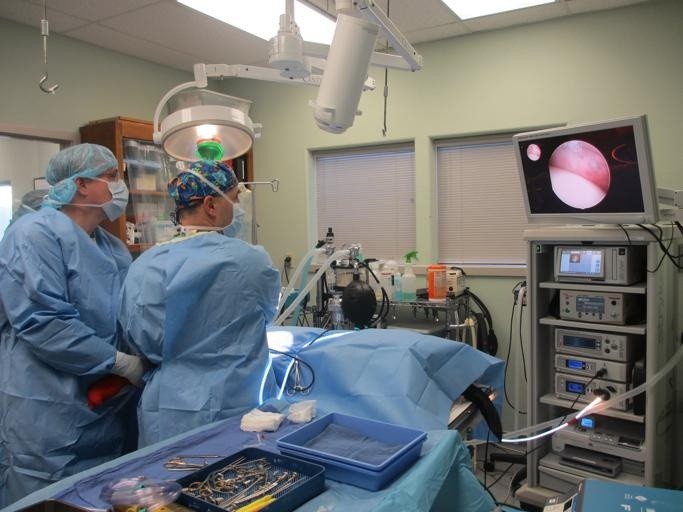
[265,324,496,441]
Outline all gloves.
[108,349,146,389]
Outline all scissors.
[164,458,204,469]
[286,355,310,396]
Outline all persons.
[114,157,284,452]
[0,140,150,512]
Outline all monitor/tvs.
[512,112,661,225]
[556,248,604,277]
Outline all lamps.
[152,63,255,164]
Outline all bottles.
[364,259,400,303]
[427,265,448,304]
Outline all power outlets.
[514,289,526,307]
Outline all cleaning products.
[401,250,420,302]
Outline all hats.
[167,159,239,210]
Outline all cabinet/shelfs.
[516,223,682,510]
[79,116,253,258]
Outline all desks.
[0,400,464,512]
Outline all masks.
[101,179,130,224]
[222,201,246,240]
